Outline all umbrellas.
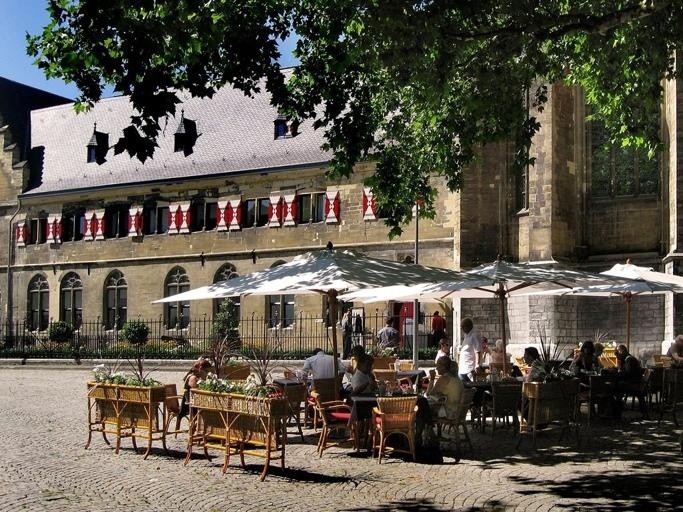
[148,241,499,438]
[554,258,682,354]
[335,253,675,380]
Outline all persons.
[177,358,209,413]
[457,317,483,385]
[430,310,446,345]
[349,354,401,445]
[341,345,376,401]
[412,337,642,445]
[339,311,352,359]
[665,334,683,361]
[415,354,463,436]
[299,347,346,429]
[375,316,400,346]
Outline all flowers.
[195,337,286,398]
[89,363,156,387]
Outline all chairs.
[161,381,207,451]
